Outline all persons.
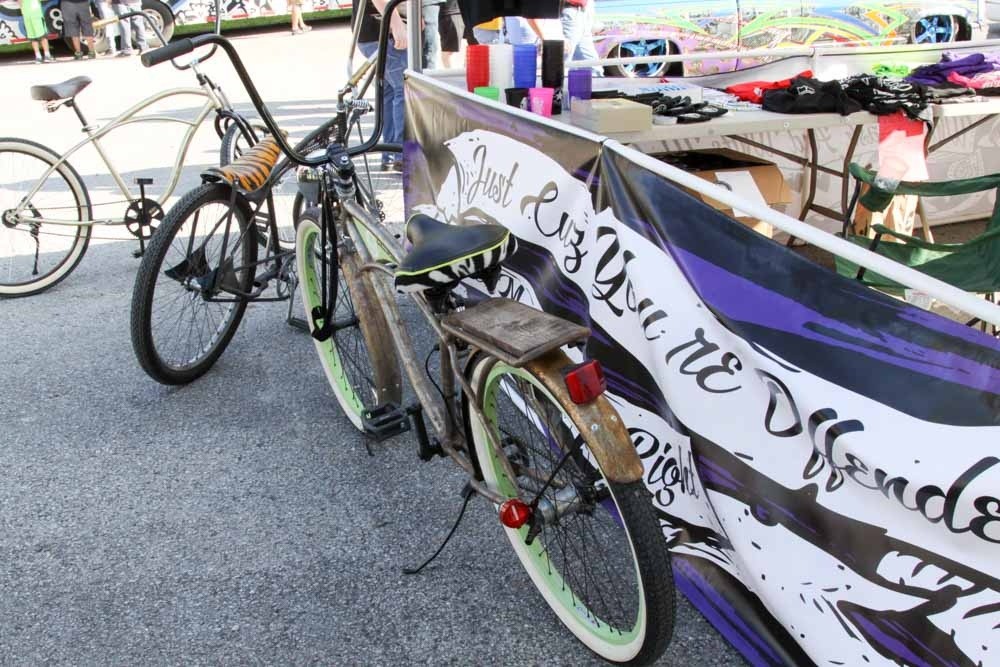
[351,0,606,173]
[285,0,313,36]
[18,0,149,64]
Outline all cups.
[465,40,565,115]
[568,70,592,101]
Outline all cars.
[0,0,354,58]
[591,0,990,81]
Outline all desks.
[614,69,1000,250]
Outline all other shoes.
[88,51,96,58]
[36,57,43,63]
[44,56,56,62]
[75,52,83,60]
[381,164,395,171]
[302,25,312,31]
[292,28,304,34]
[395,162,403,172]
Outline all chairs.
[828,164,1000,337]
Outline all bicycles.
[0,0,311,298]
[128,0,404,388]
[137,0,682,667]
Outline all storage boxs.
[571,98,652,135]
